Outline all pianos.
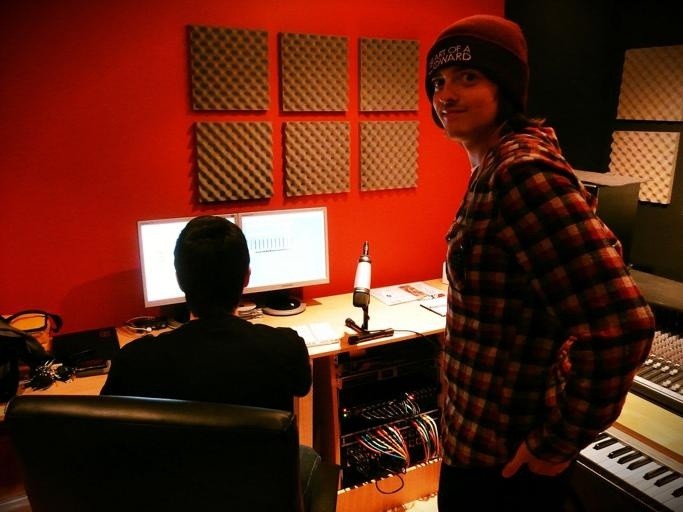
[577,430,683,511]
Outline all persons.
[423,16,656,511]
[98,215,319,499]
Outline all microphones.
[353,256,371,306]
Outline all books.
[369,282,447,307]
[286,322,341,348]
[421,296,448,318]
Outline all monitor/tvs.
[138,212,237,329]
[237,207,330,316]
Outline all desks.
[0,277,448,512]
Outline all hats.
[424,14,529,113]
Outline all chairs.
[5,395,340,512]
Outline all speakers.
[573,169,641,266]
